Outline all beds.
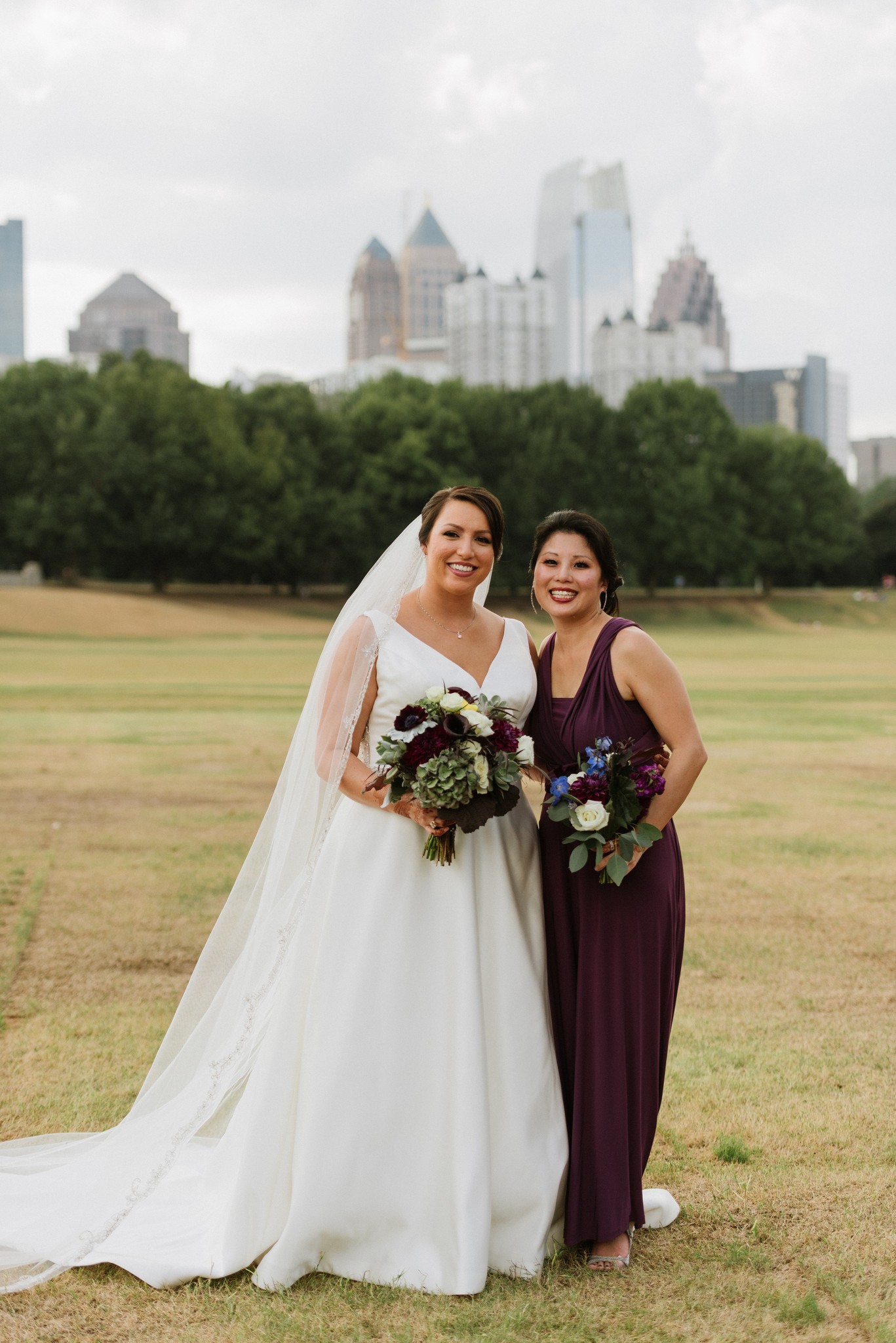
[359,679,544,866]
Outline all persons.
[0,485,571,1296]
[519,509,708,1271]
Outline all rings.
[430,816,438,829]
[608,840,614,850]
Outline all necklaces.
[417,584,476,639]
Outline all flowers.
[540,732,666,886]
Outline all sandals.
[587,1223,634,1272]
